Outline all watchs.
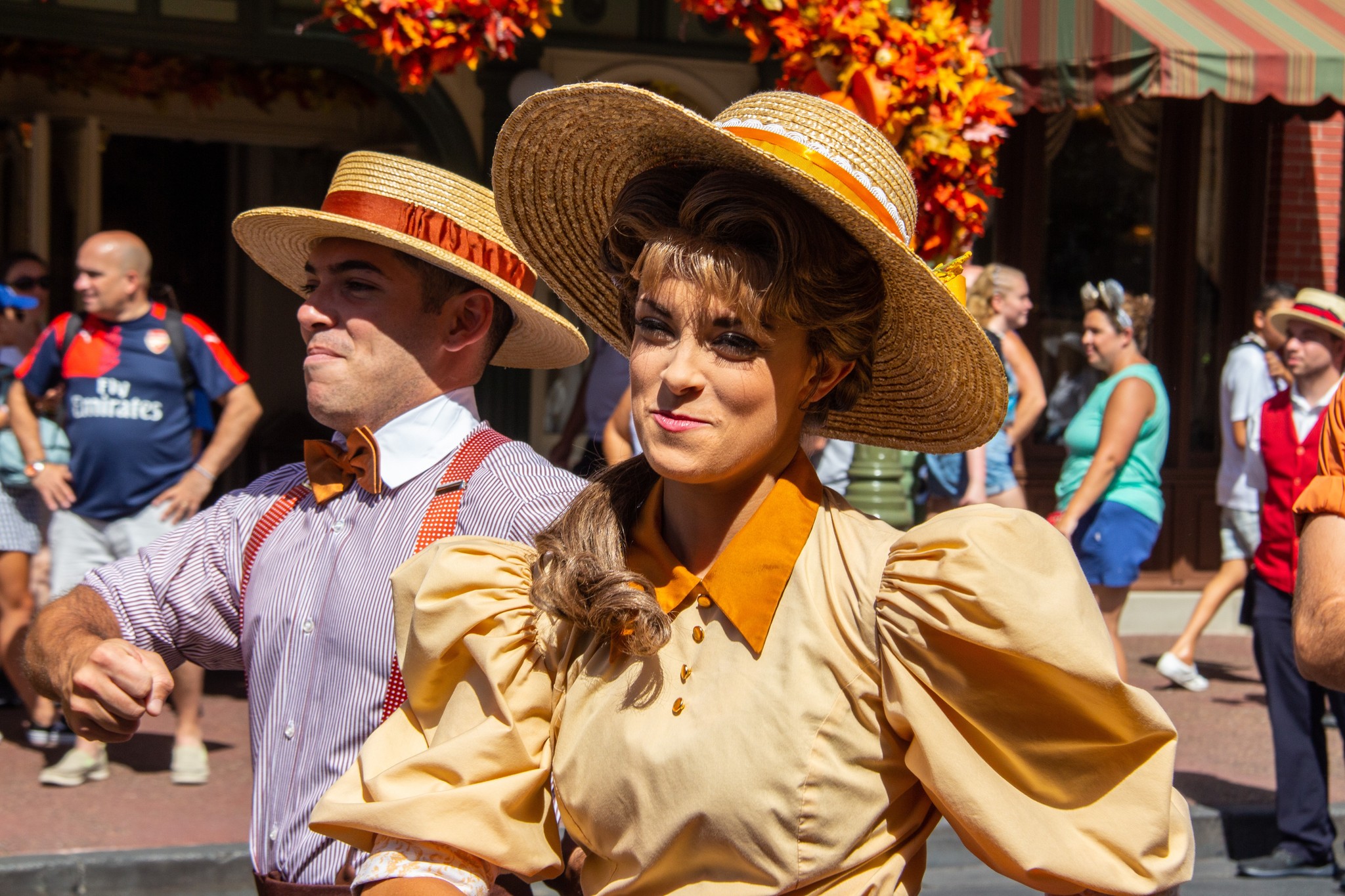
[24,457,49,479]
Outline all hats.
[302,425,381,502]
[491,78,1009,453]
[233,147,590,373]
[1268,286,1345,341]
[1041,330,1088,364]
[0,283,41,310]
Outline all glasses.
[7,274,56,292]
[15,311,24,323]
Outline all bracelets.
[193,462,215,482]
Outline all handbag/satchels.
[0,418,70,490]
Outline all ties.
[548,348,598,466]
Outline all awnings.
[975,0,1345,119]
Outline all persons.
[912,258,1048,520]
[1290,374,1345,694]
[0,284,76,748]
[1156,280,1296,692]
[799,428,855,501]
[1054,278,1169,688]
[1237,288,1345,879]
[6,228,262,787]
[0,245,59,357]
[307,74,1193,896]
[21,149,601,896]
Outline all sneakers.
[1156,648,1211,693]
[39,748,111,787]
[25,712,76,746]
[1235,834,1335,876]
[166,736,211,786]
